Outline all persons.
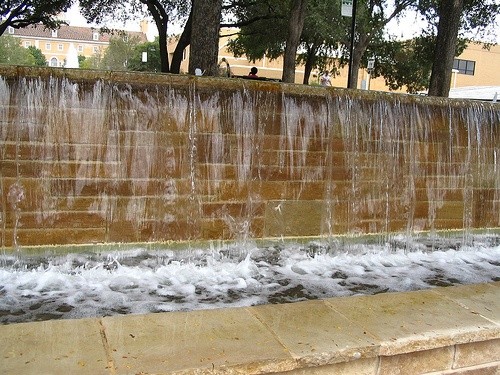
[247,67,259,77]
[320,70,332,87]
